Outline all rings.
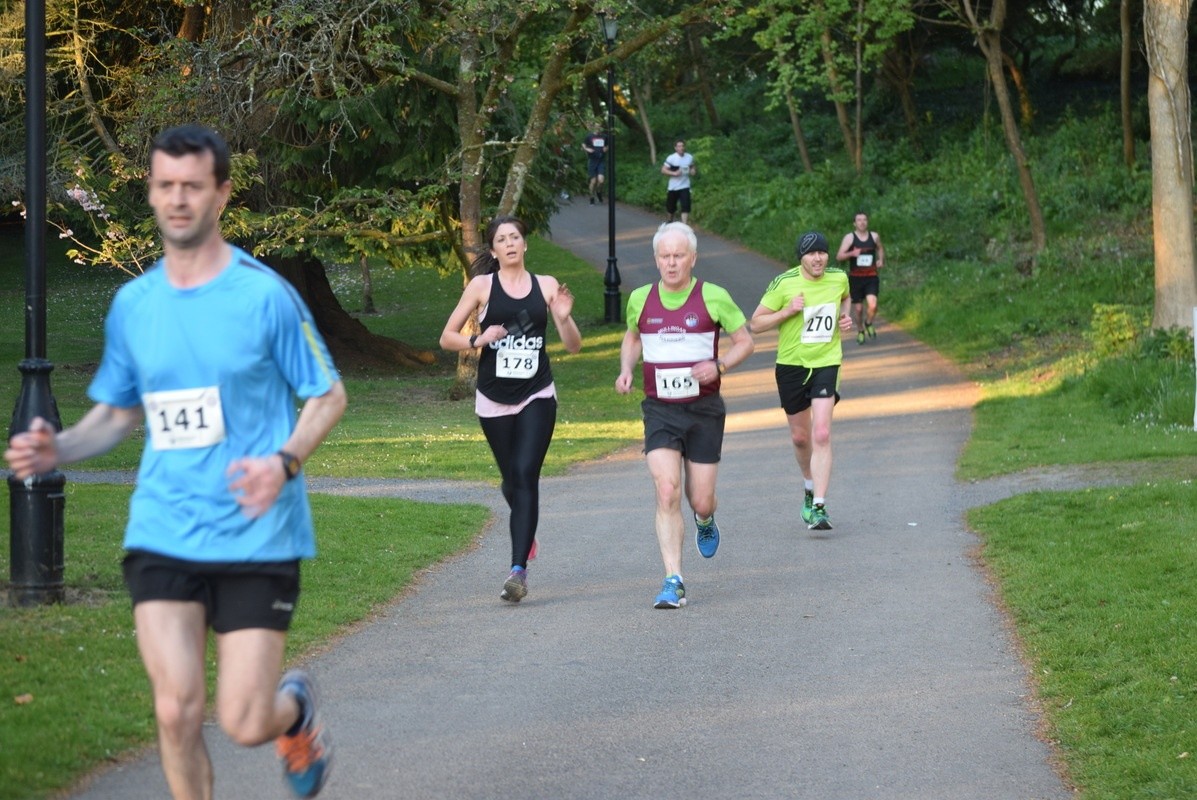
[704,373,707,376]
[704,377,707,380]
[848,326,851,330]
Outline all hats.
[797,231,828,262]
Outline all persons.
[5,126,333,800]
[438,218,583,602]
[581,122,610,207]
[661,139,696,225]
[750,233,854,530]
[549,115,575,200]
[835,212,885,345]
[614,221,755,609]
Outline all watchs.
[277,450,300,479]
[469,335,477,348]
[716,360,727,375]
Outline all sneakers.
[527,539,537,559]
[501,572,528,602]
[864,321,876,339]
[693,509,720,558]
[801,488,814,522]
[807,503,832,530]
[653,573,686,608]
[271,669,332,800]
[857,329,865,344]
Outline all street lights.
[594,5,623,325]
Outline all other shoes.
[597,192,602,201]
[590,197,595,204]
